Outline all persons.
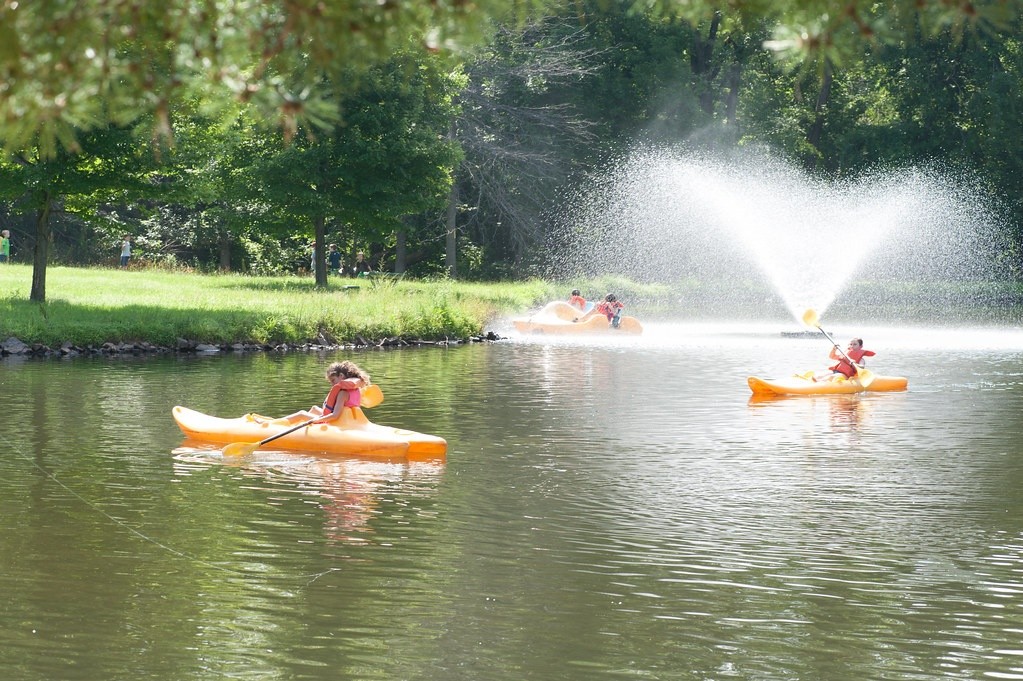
[566,290,586,312]
[571,293,621,323]
[294,242,373,279]
[812,337,866,385]
[0,229,10,265]
[119,234,131,270]
[250,360,371,424]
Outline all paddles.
[221,383,385,455]
[804,310,874,387]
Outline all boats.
[747,373,909,394]
[514,302,642,336]
[171,405,448,457]
